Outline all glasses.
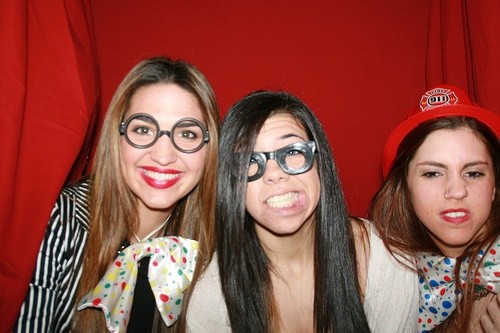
[233,140,317,182]
[118,112,210,154]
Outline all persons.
[368,84,500,333]
[183,90,420,333]
[11,57,221,333]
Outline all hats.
[381,83,500,183]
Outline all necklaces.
[131,213,172,243]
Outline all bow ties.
[76,235,200,333]
[415,234,500,331]
